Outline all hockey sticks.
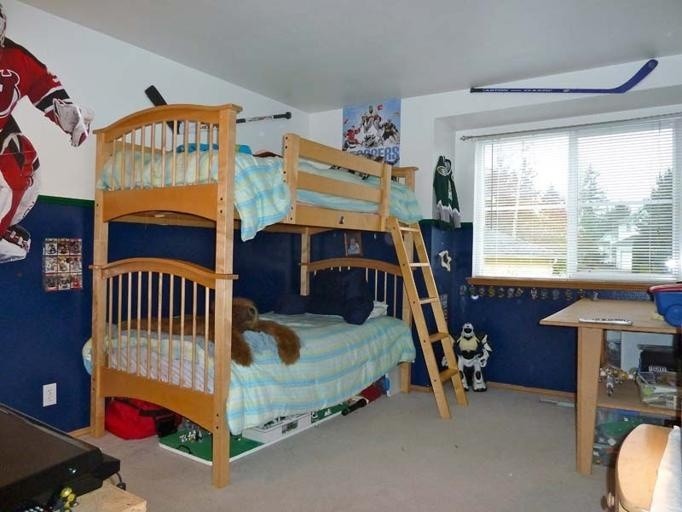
[470,59,658,93]
[145,85,291,136]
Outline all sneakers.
[0,229,31,256]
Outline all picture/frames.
[344,229,364,258]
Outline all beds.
[91,104,418,485]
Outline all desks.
[538,298,681,475]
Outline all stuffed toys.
[118,297,300,366]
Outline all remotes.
[578,317,632,326]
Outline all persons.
[347,237,360,254]
[442,322,493,392]
[0,6,90,263]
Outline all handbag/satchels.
[105,396,183,439]
